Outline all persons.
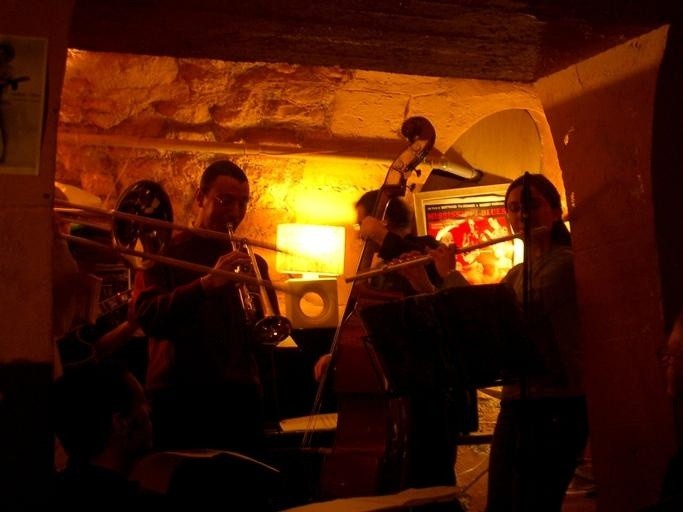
[311,188,482,512]
[439,213,512,285]
[49,362,203,509]
[373,176,589,509]
[127,160,280,510]
[652,312,681,512]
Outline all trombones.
[53,183,324,297]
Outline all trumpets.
[228,223,291,344]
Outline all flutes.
[345,226,549,284]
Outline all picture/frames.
[412,182,522,286]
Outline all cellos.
[321,116,436,498]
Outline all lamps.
[277,224,346,279]
